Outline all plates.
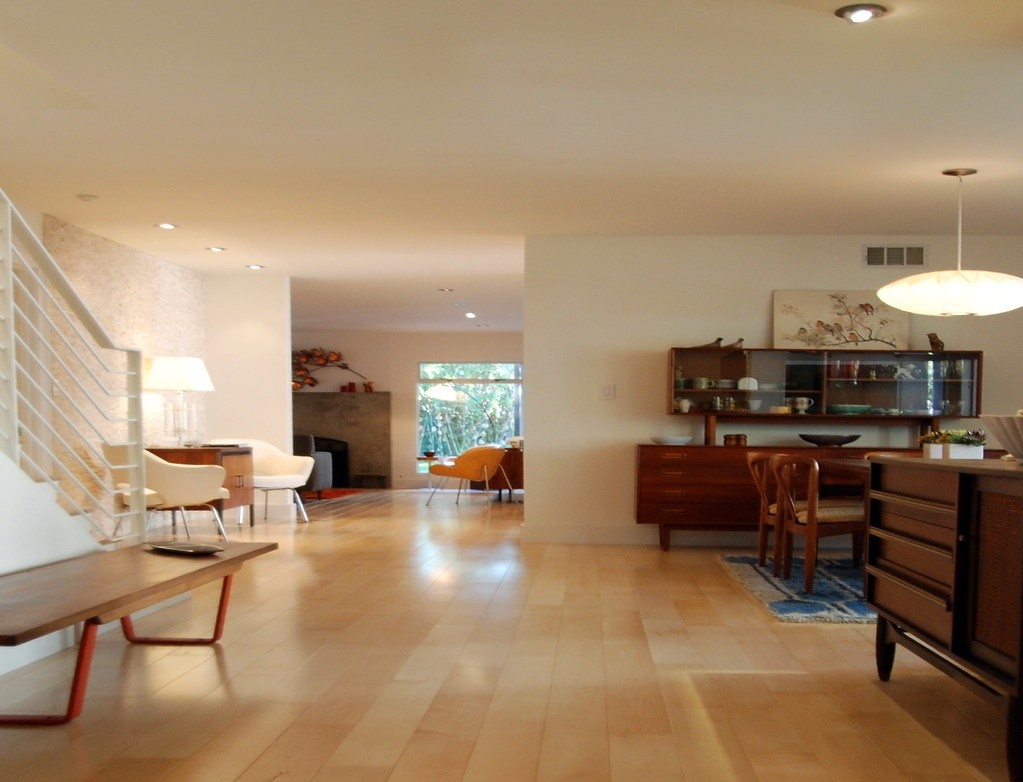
[831,404,872,415]
[648,436,693,445]
[906,409,941,415]
[151,543,225,556]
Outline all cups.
[829,360,840,377]
[847,360,859,378]
[749,399,762,410]
[889,408,903,416]
[795,398,814,414]
[696,377,714,389]
[340,385,348,392]
[349,383,357,392]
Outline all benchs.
[294,434,333,500]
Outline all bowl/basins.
[978,411,1023,465]
[422,452,436,457]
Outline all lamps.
[141,355,215,448]
[876,168,1023,317]
[424,382,501,443]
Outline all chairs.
[110,450,232,542]
[743,450,925,594]
[425,444,522,514]
[203,436,315,525]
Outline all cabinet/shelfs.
[470,448,523,504]
[862,451,1023,782]
[635,444,1008,553]
[668,347,984,419]
[147,444,255,536]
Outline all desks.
[0,539,278,727]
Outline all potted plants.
[915,430,988,459]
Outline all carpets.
[712,553,879,624]
[299,487,363,499]
